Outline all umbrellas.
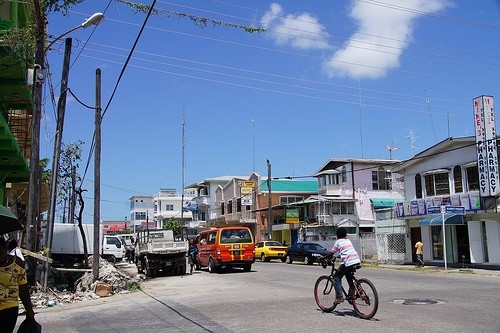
[0,205,24,236]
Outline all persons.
[188,238,198,275]
[415,238,425,267]
[0,236,36,333]
[283,240,287,246]
[320,227,364,304]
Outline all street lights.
[23,10,108,295]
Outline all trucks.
[132,229,189,278]
[50,221,126,266]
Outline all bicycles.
[312,255,379,320]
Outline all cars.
[285,241,335,266]
[255,240,288,263]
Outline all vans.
[119,234,134,250]
[193,225,256,273]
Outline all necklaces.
[3,255,9,267]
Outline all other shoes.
[422,263,424,267]
[334,297,344,304]
[346,294,356,299]
